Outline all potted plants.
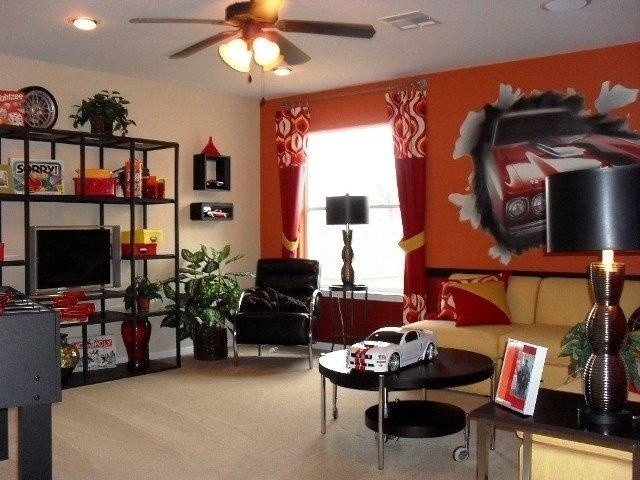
[69,90,137,136]
[160,243,256,362]
[124,275,163,313]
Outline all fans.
[130,0,376,66]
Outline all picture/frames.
[495,338,549,417]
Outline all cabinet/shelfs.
[190,203,234,222]
[0,123,181,390]
[193,153,231,192]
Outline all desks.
[329,286,368,352]
[467,376,640,480]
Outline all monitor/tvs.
[27,224,120,300]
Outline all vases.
[60,333,79,378]
[121,317,152,366]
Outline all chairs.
[233,258,324,369]
[495,277,639,404]
[399,273,542,404]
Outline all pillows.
[434,270,512,321]
[449,280,513,327]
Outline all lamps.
[540,0,591,12]
[325,193,370,287]
[546,163,640,416]
[218,37,285,73]
[67,15,99,30]
